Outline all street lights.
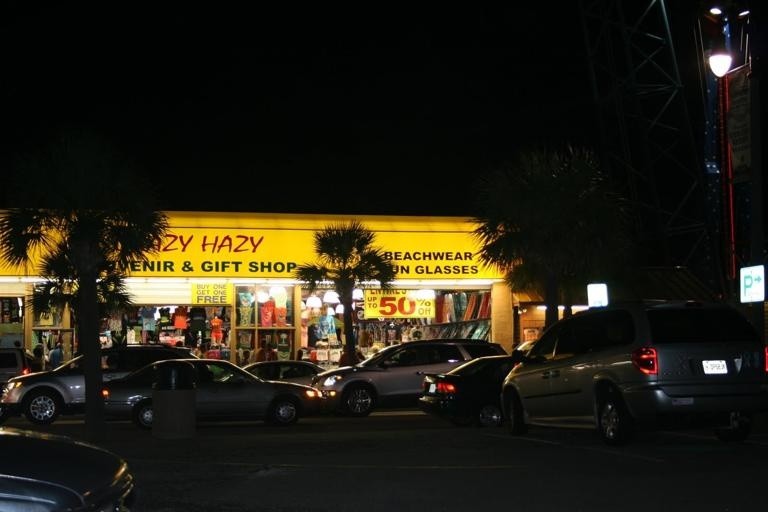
[706,5,743,302]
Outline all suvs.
[0,344,214,425]
[1,346,31,424]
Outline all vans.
[311,339,508,417]
[501,301,768,448]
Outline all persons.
[255,339,266,362]
[240,350,250,366]
[197,343,208,359]
[268,342,278,360]
[371,346,379,353]
[13,336,65,373]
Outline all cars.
[102,359,323,432]
[0,426,136,512]
[511,336,537,354]
[418,354,521,433]
[238,359,326,387]
